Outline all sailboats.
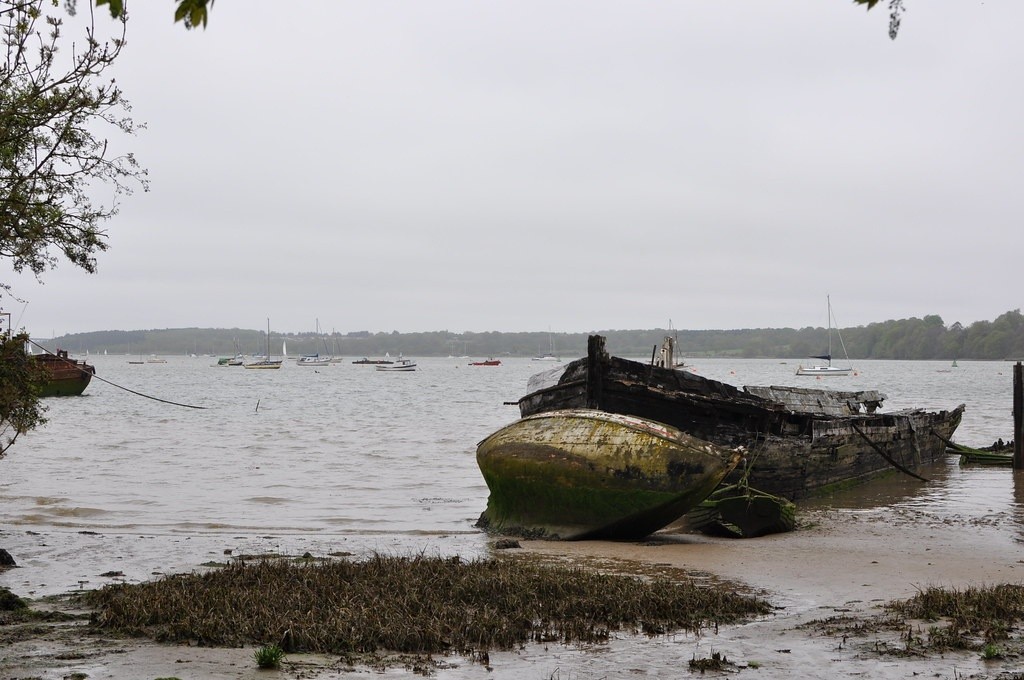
[531,327,559,361]
[297,318,345,366]
[243,318,283,369]
[796,294,853,376]
[655,319,685,368]
[218,336,242,365]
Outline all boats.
[475,409,744,540]
[147,358,168,364]
[518,334,965,502]
[352,358,394,364]
[467,360,500,365]
[686,483,796,539]
[0,313,95,396]
[129,361,144,364]
[376,360,417,371]
